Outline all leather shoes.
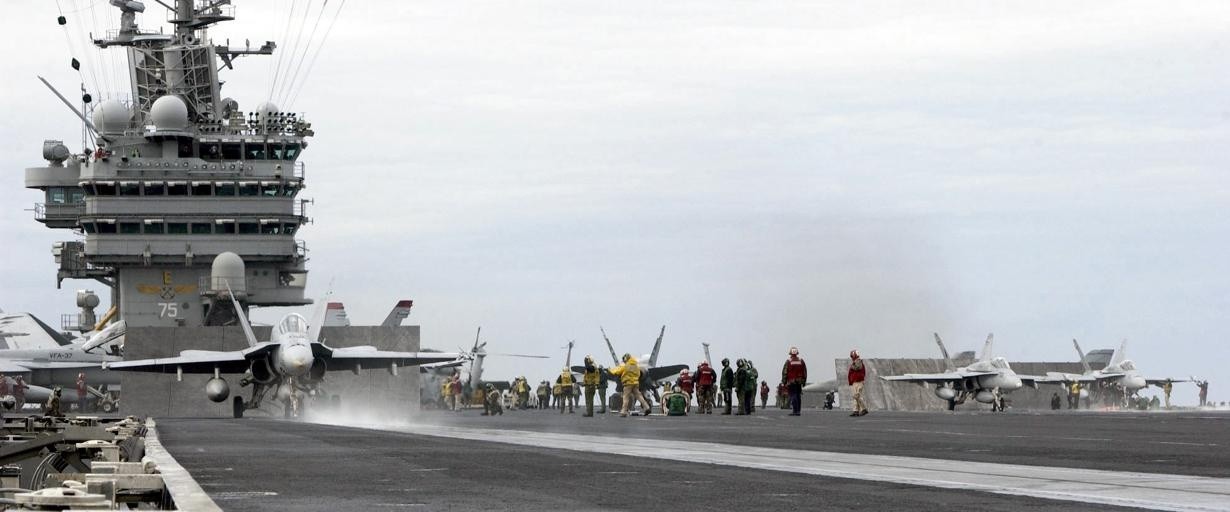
[582,414,593,417]
[480,412,489,415]
[850,412,859,416]
[860,409,868,416]
[644,409,651,416]
[597,411,605,413]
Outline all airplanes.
[103,278,467,419]
[559,325,713,412]
[878,332,1096,412]
[0,310,128,414]
[1045,339,1202,410]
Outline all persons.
[76,372,88,413]
[1196,381,1208,407]
[1130,396,1160,410]
[823,390,835,410]
[15,376,30,413]
[1162,381,1172,409]
[442,347,808,419]
[0,375,8,396]
[848,349,868,417]
[44,386,65,421]
[1050,380,1081,410]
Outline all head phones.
[724,359,728,365]
[739,359,742,366]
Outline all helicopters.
[418,326,552,412]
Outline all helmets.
[680,358,752,375]
[674,386,681,392]
[790,347,799,355]
[486,375,550,392]
[850,350,859,360]
[563,366,570,373]
[622,353,631,363]
[585,356,592,364]
